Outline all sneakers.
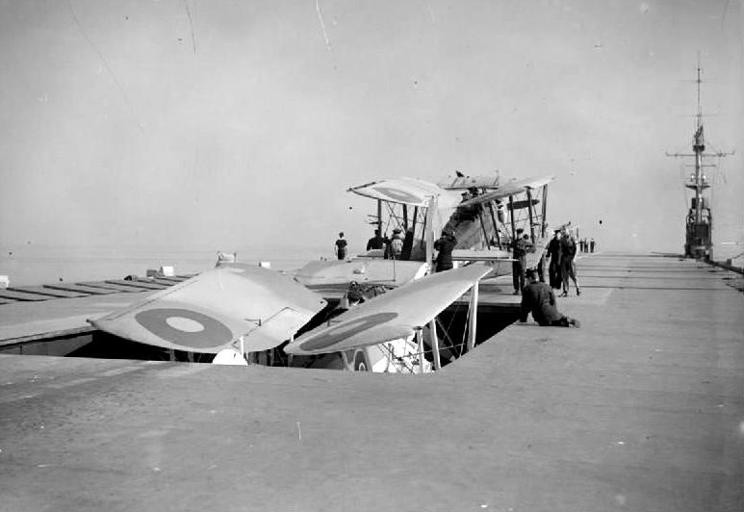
[565,317,581,328]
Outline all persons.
[433,229,457,273]
[367,228,413,259]
[505,227,596,328]
[334,231,348,260]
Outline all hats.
[374,229,379,234]
[554,229,561,233]
[516,228,524,232]
[391,229,401,234]
